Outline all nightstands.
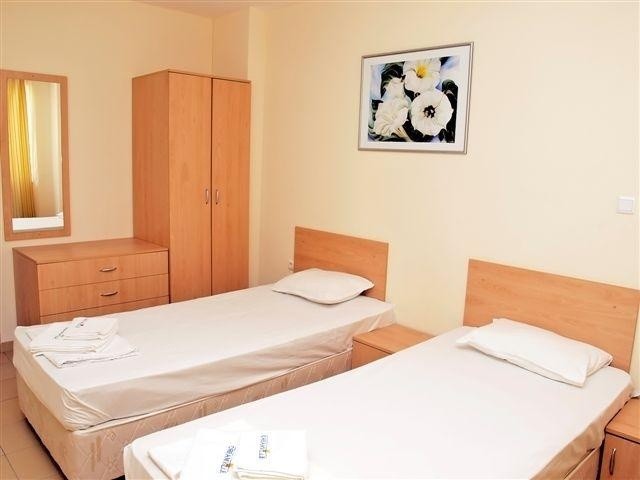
[599,398,640,480]
[349,323,437,368]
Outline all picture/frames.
[357,41,475,155]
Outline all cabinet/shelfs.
[131,68,252,305]
[12,237,171,326]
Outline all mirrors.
[1,69,72,241]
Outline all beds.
[12,226,389,480]
[120,258,639,480]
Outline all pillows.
[454,317,614,388]
[269,267,376,305]
